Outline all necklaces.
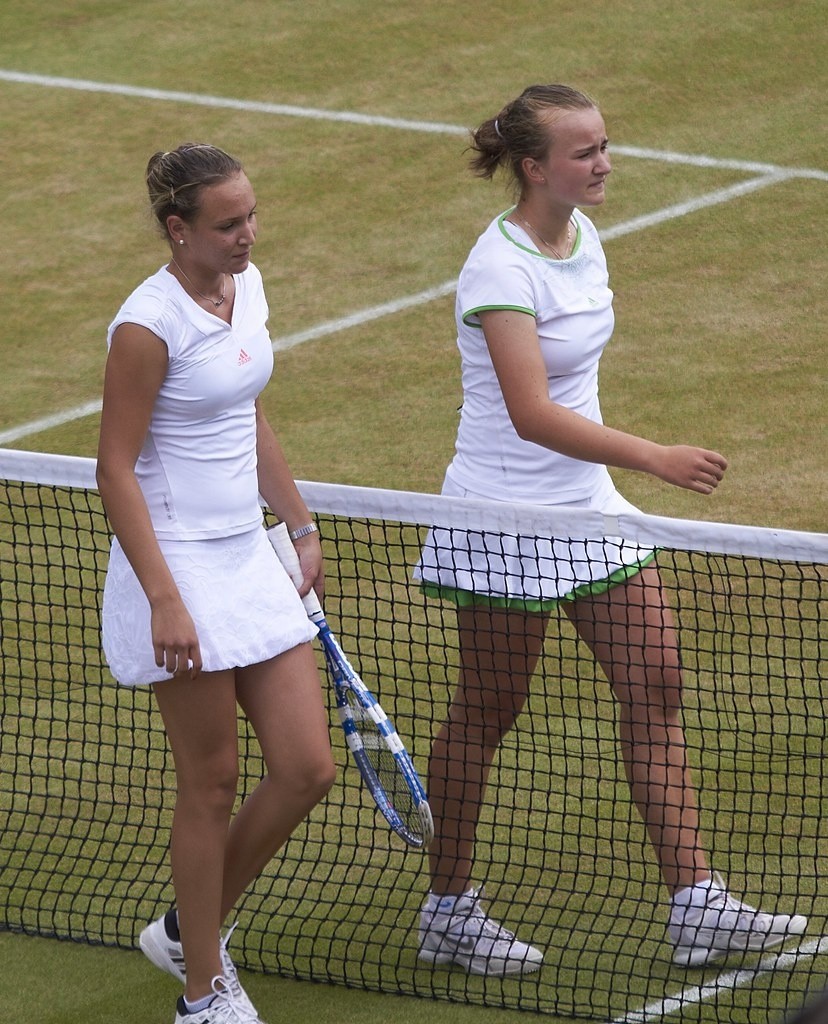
[170,255,228,308]
[514,208,574,260]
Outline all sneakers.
[416,887,543,976]
[669,869,807,969]
[173,975,265,1024]
[138,908,259,1016]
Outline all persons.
[411,83,806,976]
[95,140,338,1024]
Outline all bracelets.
[289,524,318,540]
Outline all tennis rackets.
[266,518,433,850]
